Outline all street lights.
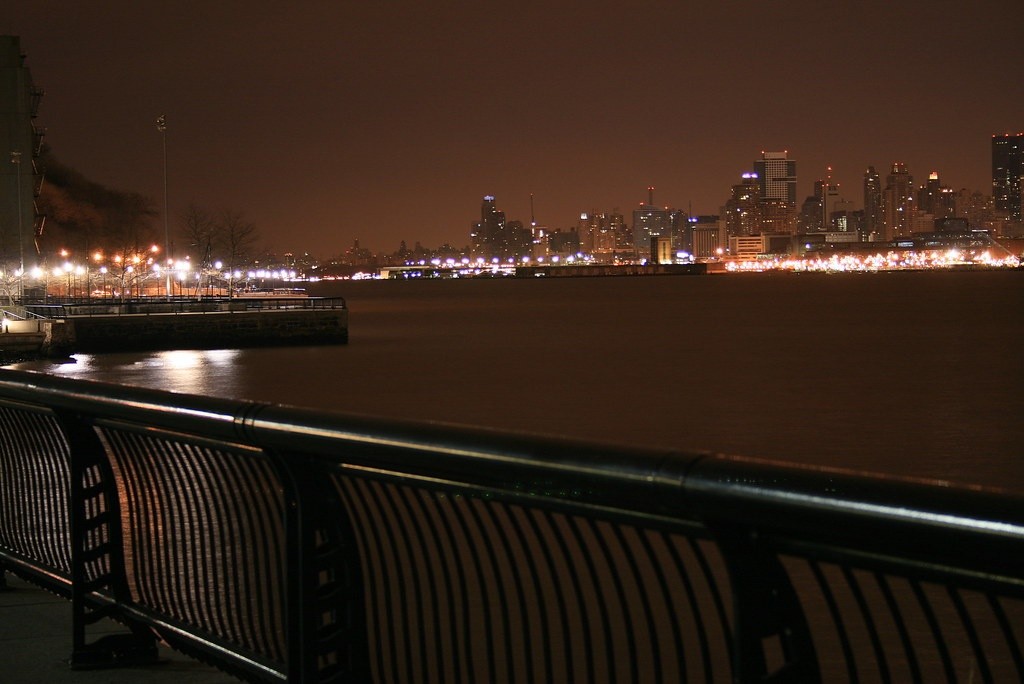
[154,263,160,295]
[75,267,84,297]
[215,261,222,300]
[64,262,73,297]
[127,266,134,299]
[14,269,20,301]
[100,267,108,298]
